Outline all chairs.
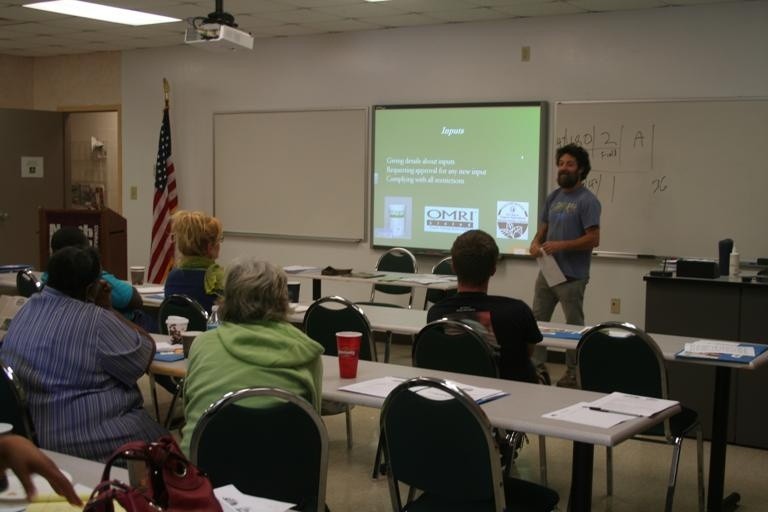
[423,256,458,311]
[353,247,418,363]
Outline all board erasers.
[757,258,768,265]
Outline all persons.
[0,422,84,507]
[427,230,551,385]
[179,261,325,461]
[0,245,173,470]
[41,228,182,397]
[165,210,225,316]
[529,143,601,391]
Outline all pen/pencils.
[589,407,643,417]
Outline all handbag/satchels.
[82,432,225,512]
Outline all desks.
[0,265,768,510]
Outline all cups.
[335,331,361,379]
[181,332,202,359]
[287,282,300,303]
[167,315,189,345]
[130,266,145,286]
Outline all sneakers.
[556,377,579,389]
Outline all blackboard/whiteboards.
[211,107,370,244]
[549,96,768,268]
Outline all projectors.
[184,23,254,52]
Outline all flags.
[147,99,179,284]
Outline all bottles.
[729,246,739,277]
[205,305,219,331]
